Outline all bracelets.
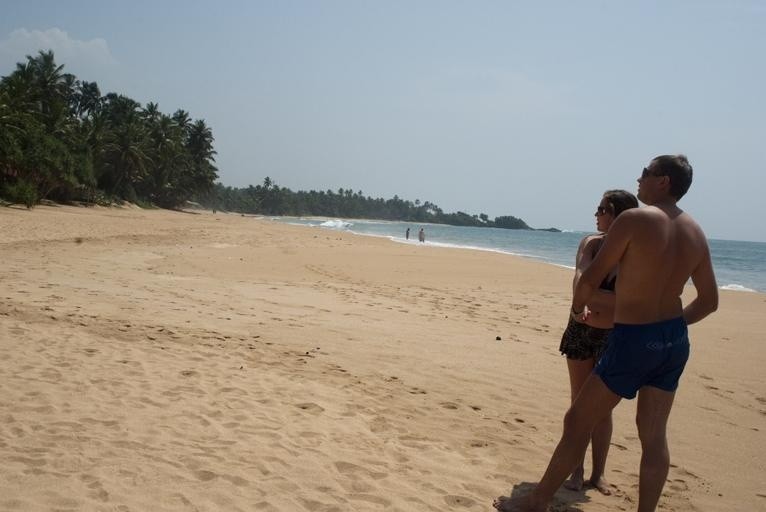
[572,305,584,318]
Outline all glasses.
[595,206,612,217]
[642,168,665,179]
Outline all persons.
[406,228,410,239]
[492,156,718,512]
[419,228,425,242]
[564,190,638,494]
